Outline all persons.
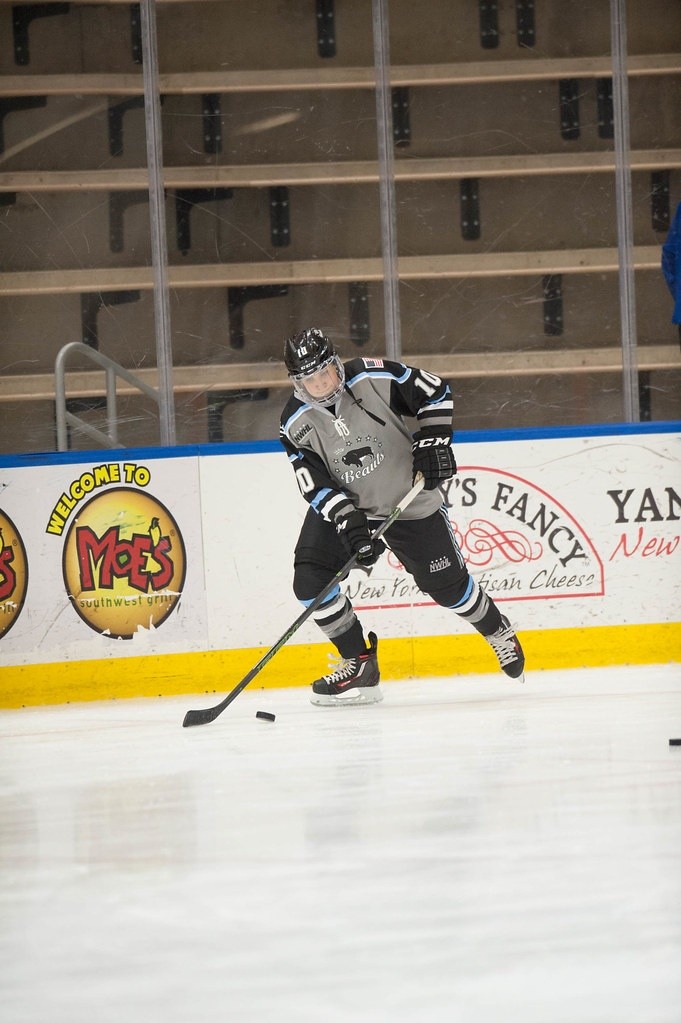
[279,326,524,706]
[661,200,681,343]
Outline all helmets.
[284,327,345,407]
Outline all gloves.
[411,428,457,490]
[334,511,385,566]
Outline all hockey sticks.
[155,476,428,729]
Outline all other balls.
[668,738,680,745]
[257,711,275,721]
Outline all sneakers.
[309,631,384,706]
[484,614,527,684]
